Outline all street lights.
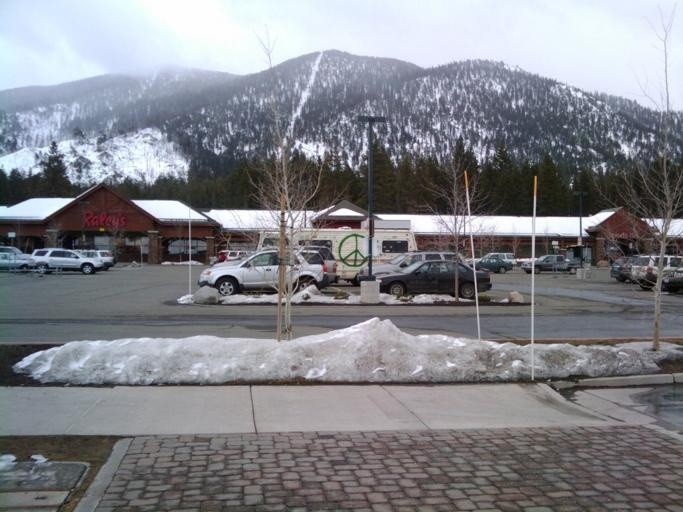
[573,191,588,245]
[358,116,387,275]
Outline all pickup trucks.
[521,254,580,274]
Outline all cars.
[0,245,116,275]
[610,254,683,293]
